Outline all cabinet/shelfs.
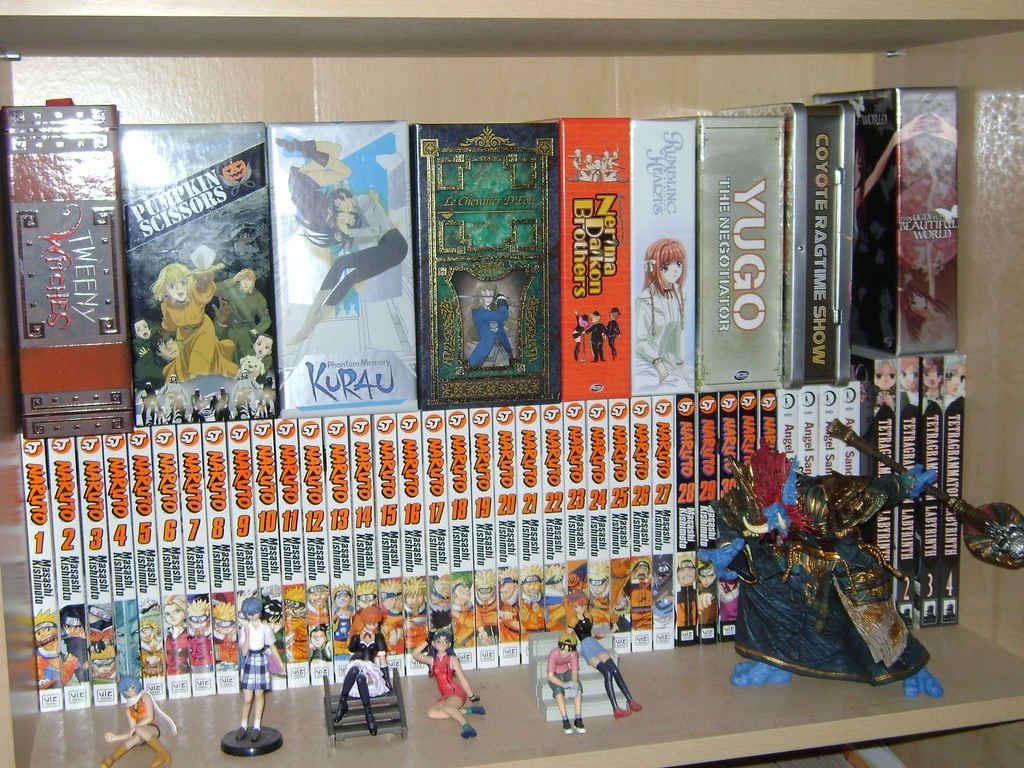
[0,0,1024,768]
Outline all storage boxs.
[0,84,962,438]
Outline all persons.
[100,674,172,768]
[546,632,587,735]
[412,628,487,739]
[696,438,945,698]
[567,590,641,719]
[333,605,394,736]
[235,596,287,743]
